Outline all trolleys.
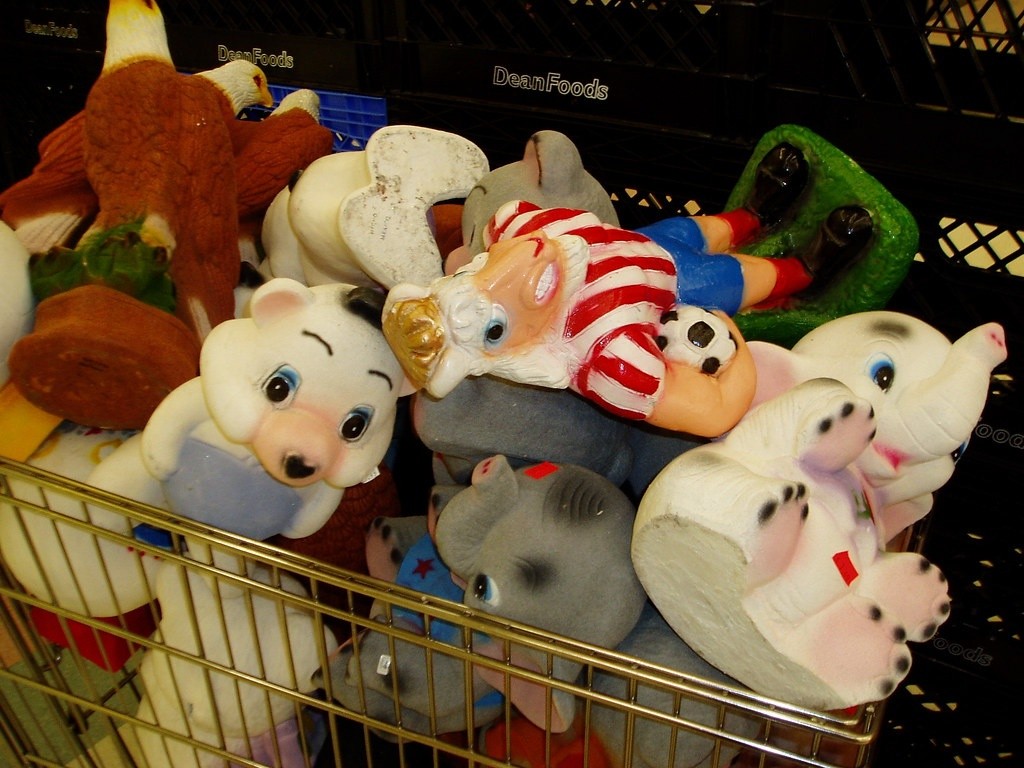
[0,446,921,768]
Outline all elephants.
[316,454,648,737]
[632,309,1008,708]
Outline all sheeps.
[385,226,591,390]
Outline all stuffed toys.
[0,0,1008,768]
